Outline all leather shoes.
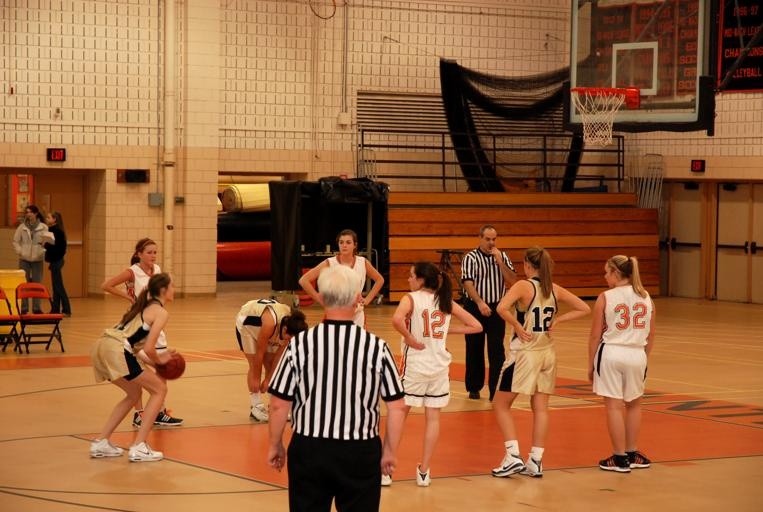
[468,391,481,399]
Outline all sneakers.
[598,454,632,473]
[153,408,184,426]
[132,410,145,429]
[89,437,125,459]
[380,474,392,486]
[491,453,526,478]
[625,451,651,469]
[519,456,543,477]
[127,441,163,462]
[248,403,269,424]
[416,463,431,487]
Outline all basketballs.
[155,349,185,380]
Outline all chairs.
[0,286,24,354]
[14,284,66,354]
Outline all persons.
[462,225,518,401]
[14,206,48,314]
[298,230,384,329]
[91,273,178,462]
[492,245,592,476]
[589,255,656,472]
[381,263,484,486]
[103,237,185,428]
[268,265,406,512]
[44,212,72,317]
[235,299,309,421]
